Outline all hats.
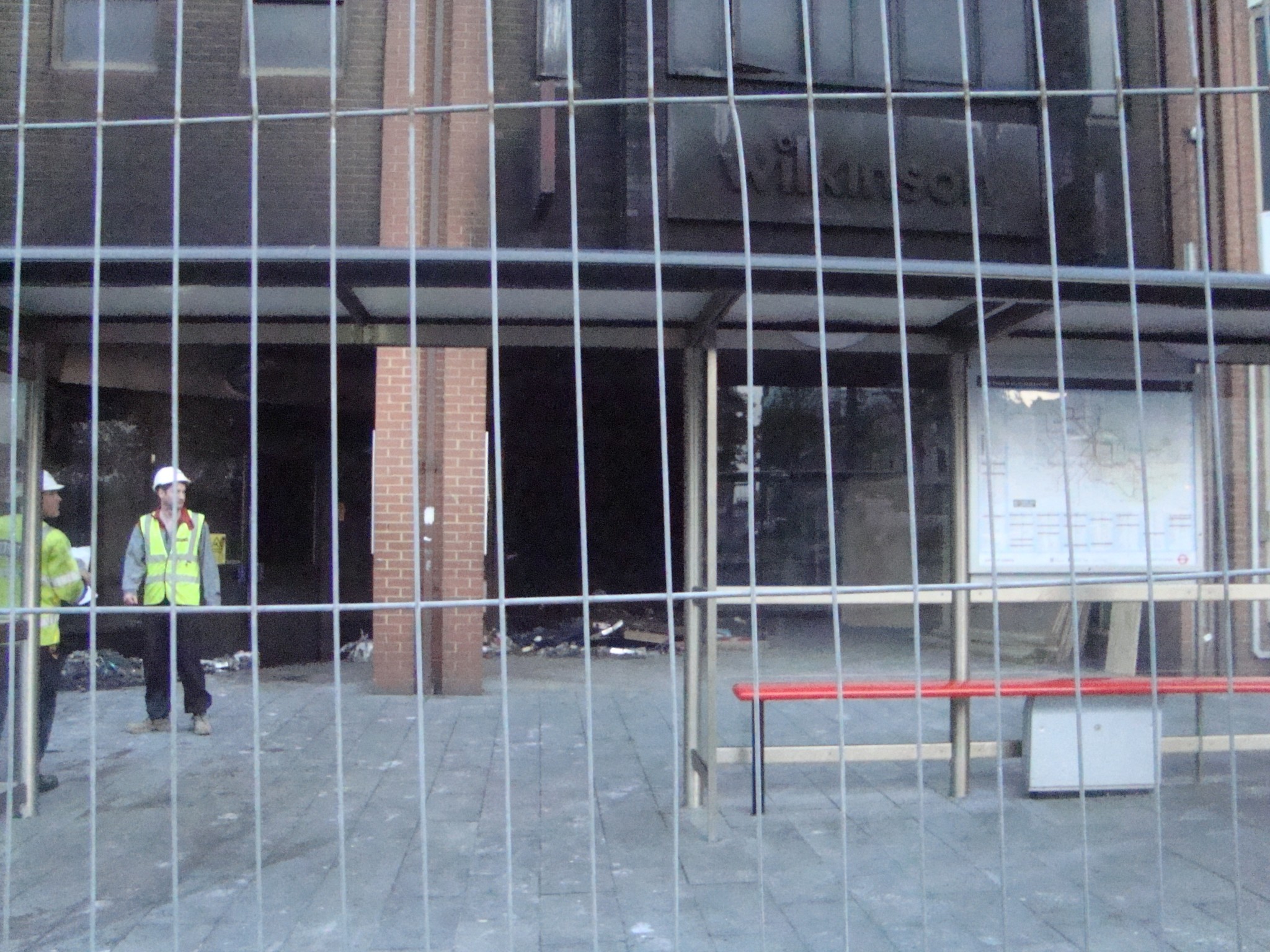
[153,467,191,491]
[39,469,64,491]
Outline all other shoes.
[192,713,211,734]
[39,773,59,793]
[125,714,170,734]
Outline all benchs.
[732,673,1269,816]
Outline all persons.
[121,465,223,738]
[0,466,96,792]
